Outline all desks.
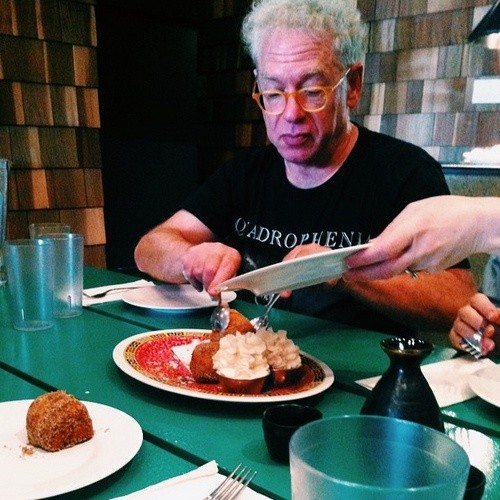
[0,266,500,500]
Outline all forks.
[203,463,257,500]
[83,287,139,299]
[459,319,489,359]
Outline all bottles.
[361,337,446,434]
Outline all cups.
[263,404,323,466]
[3,239,55,331]
[289,415,470,500]
[29,223,70,239]
[34,233,83,318]
[463,465,486,500]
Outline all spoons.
[250,294,280,331]
[211,292,230,332]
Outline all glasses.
[252,68,351,116]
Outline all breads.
[189,308,260,384]
[26,389,95,451]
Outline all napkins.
[354,356,496,411]
[59,276,156,308]
[110,461,271,500]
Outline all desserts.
[213,327,302,393]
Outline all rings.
[405,268,416,279]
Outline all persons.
[342,195,500,281]
[448,254,500,356]
[134,0,477,335]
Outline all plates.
[0,399,143,500]
[113,329,335,402]
[469,364,500,408]
[122,284,237,313]
[219,242,372,296]
